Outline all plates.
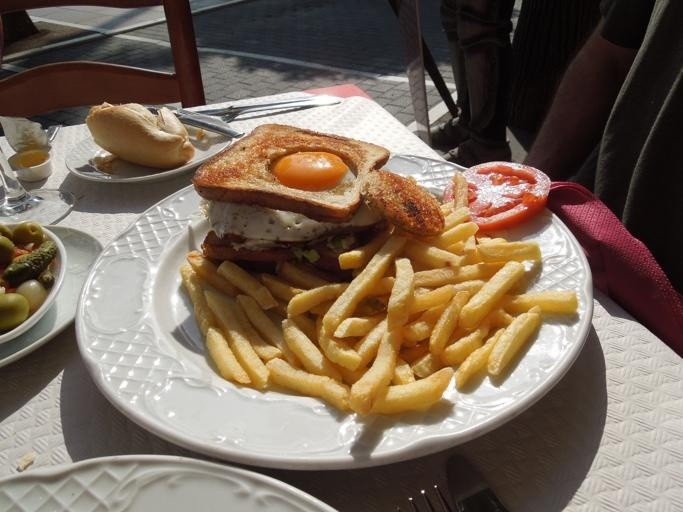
[0,226,101,367]
[0,228,65,346]
[75,155,595,470]
[64,114,234,183]
[0,453,337,510]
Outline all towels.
[547,180,683,357]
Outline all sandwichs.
[191,122,443,272]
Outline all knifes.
[148,106,240,138]
[198,95,342,120]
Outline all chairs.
[1,0,205,137]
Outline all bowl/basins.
[5,149,55,183]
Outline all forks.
[396,484,453,511]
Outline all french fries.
[178,170,579,421]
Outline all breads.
[86,98,195,169]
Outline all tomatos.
[443,159,552,232]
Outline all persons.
[426,1,514,167]
[502,0,606,136]
[523,0,682,358]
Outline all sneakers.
[411,111,511,167]
